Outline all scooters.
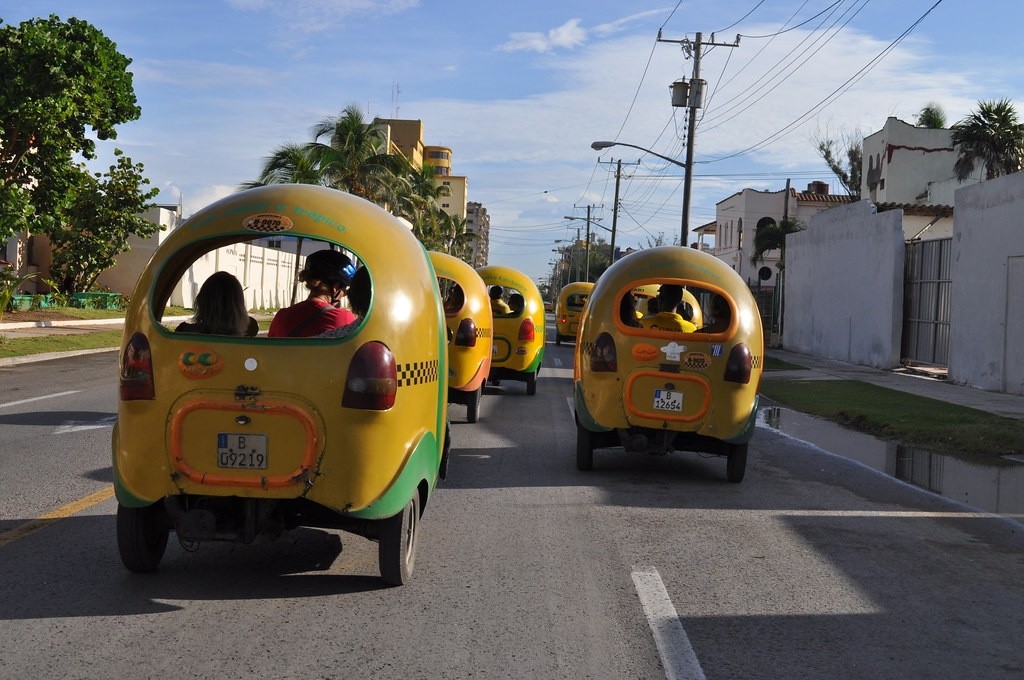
[474,265,546,396]
[109,184,450,589]
[556,281,595,346]
[631,284,704,329]
[573,246,764,484]
[427,250,495,423]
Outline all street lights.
[564,216,618,265]
[554,239,590,282]
[591,140,694,246]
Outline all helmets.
[656,284,683,302]
[299,250,356,284]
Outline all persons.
[267,250,372,337]
[445,284,464,311]
[622,283,729,333]
[489,286,524,316]
[175,271,259,336]
